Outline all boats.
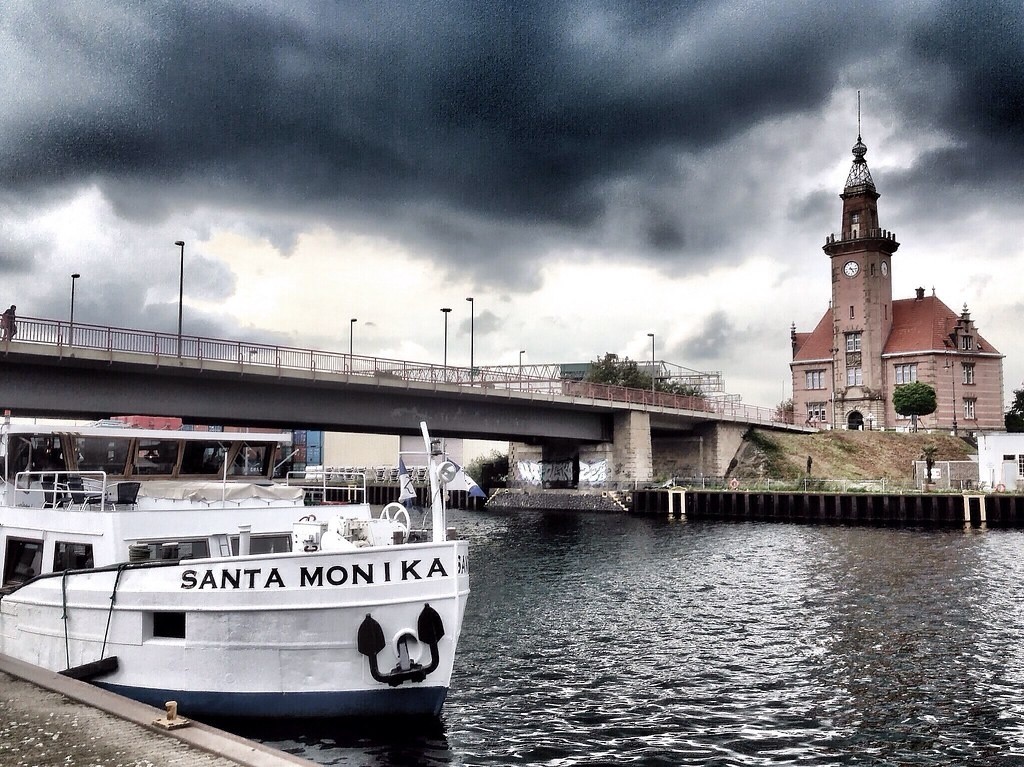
[0,409,472,724]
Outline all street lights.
[519,350,525,392]
[465,297,475,387]
[174,240,185,357]
[440,308,452,383]
[647,332,655,406]
[68,273,81,346]
[350,318,358,375]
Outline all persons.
[806,455,813,474]
[1,305,18,341]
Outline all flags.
[397,457,417,504]
[445,460,486,498]
